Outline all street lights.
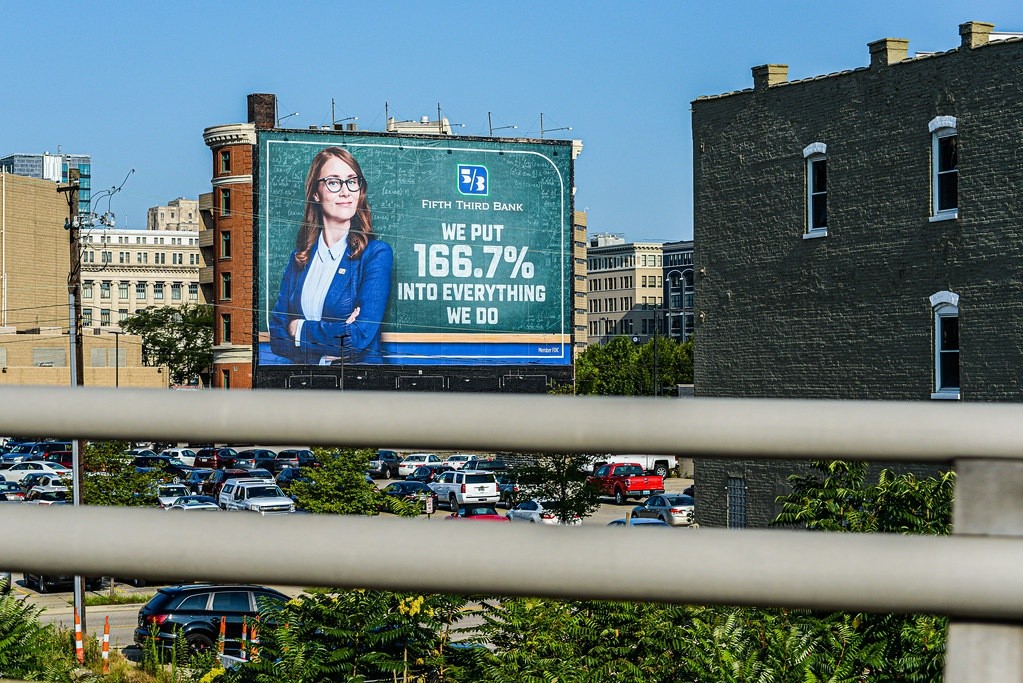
[666,269,693,344]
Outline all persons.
[269,147,393,365]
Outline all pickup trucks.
[584,461,665,506]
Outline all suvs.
[427,469,501,512]
[133,582,340,669]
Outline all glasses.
[317,177,363,193]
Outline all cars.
[379,481,439,515]
[0,441,542,513]
[505,497,583,526]
[444,502,510,522]
[332,623,486,678]
[607,517,673,527]
[631,485,694,527]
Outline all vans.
[576,454,679,481]
